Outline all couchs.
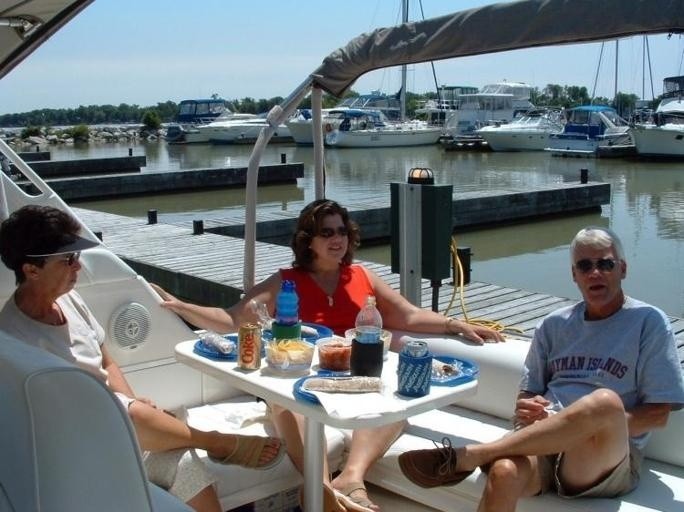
[1,328,684,512]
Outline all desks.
[174,328,479,512]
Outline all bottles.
[275,280,298,326]
[354,296,383,376]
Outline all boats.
[543,105,619,158]
[614,75,683,162]
[477,107,568,153]
[159,96,401,144]
[596,106,655,158]
[438,119,512,152]
[413,81,539,129]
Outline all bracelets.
[444,316,458,334]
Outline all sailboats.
[283,2,446,147]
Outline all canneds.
[402,341,427,357]
[238,321,263,371]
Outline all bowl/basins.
[263,340,314,369]
[345,327,392,362]
[315,335,352,370]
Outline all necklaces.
[313,271,339,293]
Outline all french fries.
[267,338,313,366]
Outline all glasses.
[319,227,348,238]
[574,259,615,272]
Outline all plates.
[430,353,478,384]
[316,368,350,376]
[264,322,332,344]
[292,375,357,405]
[193,335,268,360]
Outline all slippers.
[298,480,380,512]
[207,431,286,470]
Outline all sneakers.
[398,436,475,489]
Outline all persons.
[0,203,286,512]
[146,197,506,512]
[398,223,684,512]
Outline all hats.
[26,232,99,257]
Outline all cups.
[350,339,383,377]
[397,349,433,398]
[272,321,302,342]
